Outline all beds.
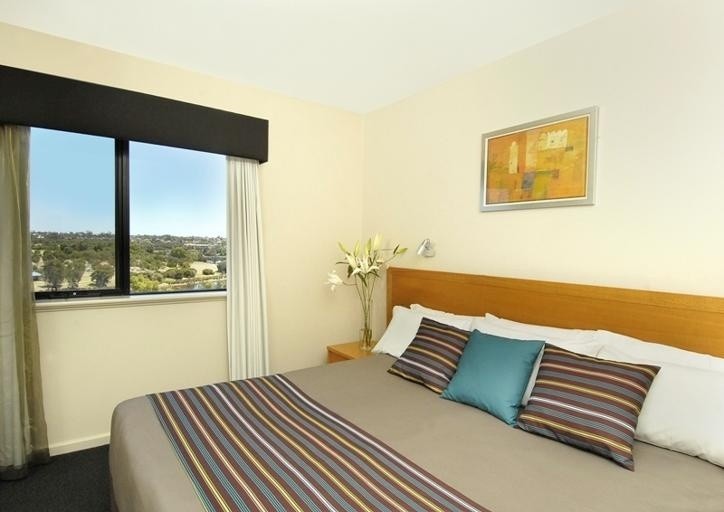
[113,264,722,511]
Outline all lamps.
[417,238,434,259]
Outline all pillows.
[373,305,477,372]
[593,334,724,468]
[480,314,604,407]
[386,316,471,397]
[442,329,544,422]
[517,345,662,470]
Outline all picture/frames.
[479,106,598,214]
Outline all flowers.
[324,232,409,347]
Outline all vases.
[361,300,372,351]
